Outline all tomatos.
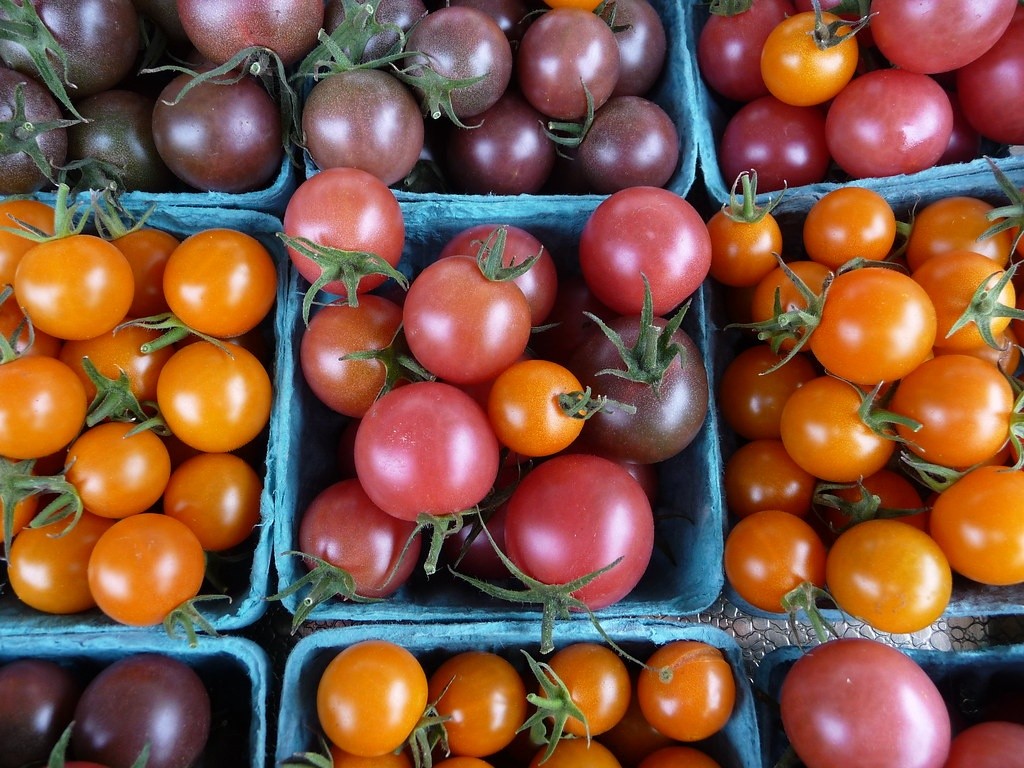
[0,0,1024,768]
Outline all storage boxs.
[2,2,1024,768]
